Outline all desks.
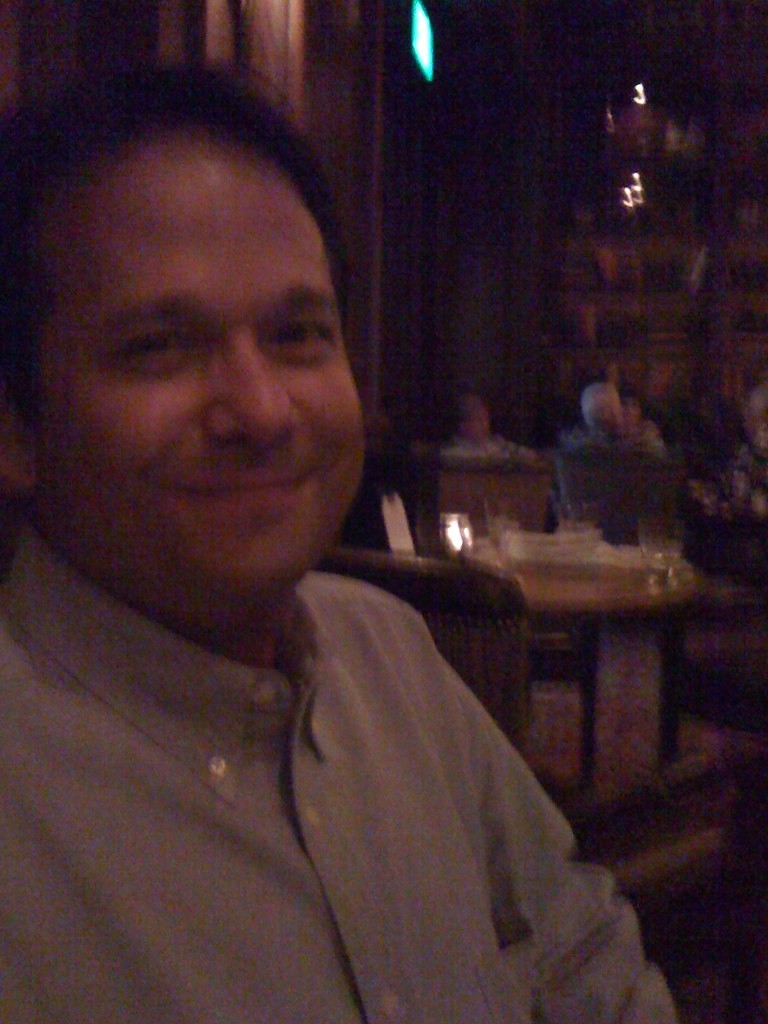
[481,562,701,780]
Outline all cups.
[639,511,687,583]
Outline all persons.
[450,382,768,588]
[0,54,679,1024]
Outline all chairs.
[361,449,670,803]
